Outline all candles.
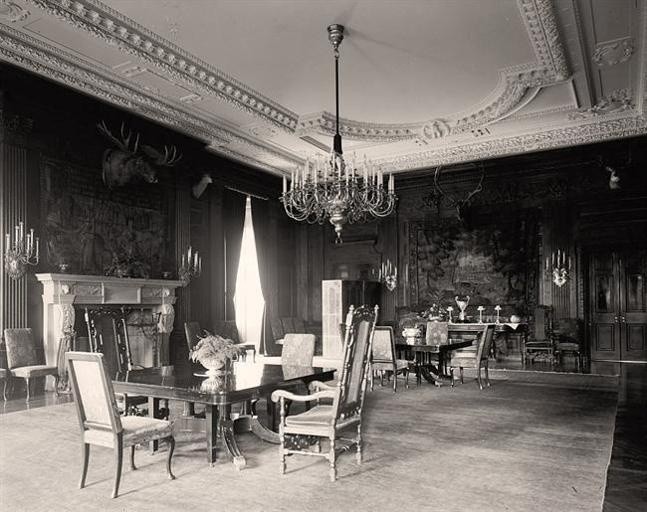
[181,247,201,272]
[372,259,397,283]
[6,221,39,256]
[545,249,572,271]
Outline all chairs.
[451,325,496,390]
[271,304,379,482]
[0,306,583,497]
[282,333,316,367]
[556,319,581,371]
[4,328,60,401]
[184,321,201,349]
[369,326,409,393]
[65,352,175,499]
[426,321,448,345]
[85,305,168,420]
[527,308,554,370]
[1,369,8,401]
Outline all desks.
[449,323,526,367]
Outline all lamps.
[282,24,399,247]
